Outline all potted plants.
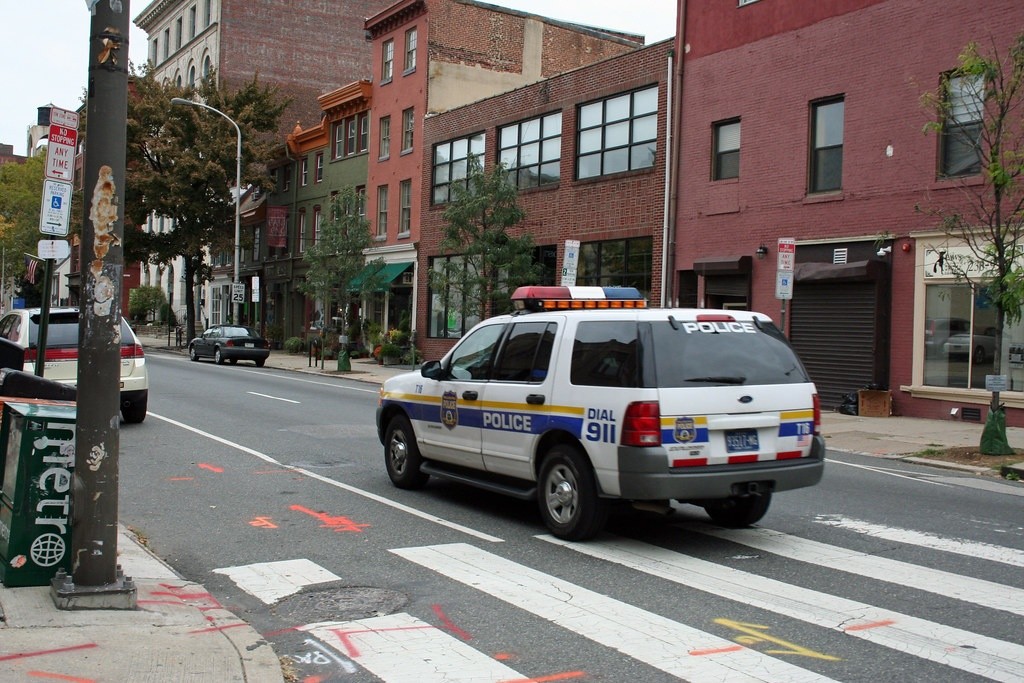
[283,322,424,367]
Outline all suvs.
[375,285,827,544]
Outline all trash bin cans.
[0,368,79,590]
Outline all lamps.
[756,243,767,260]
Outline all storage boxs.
[857,389,892,417]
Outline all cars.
[0,305,151,424]
[188,326,269,367]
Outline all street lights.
[170,97,242,326]
